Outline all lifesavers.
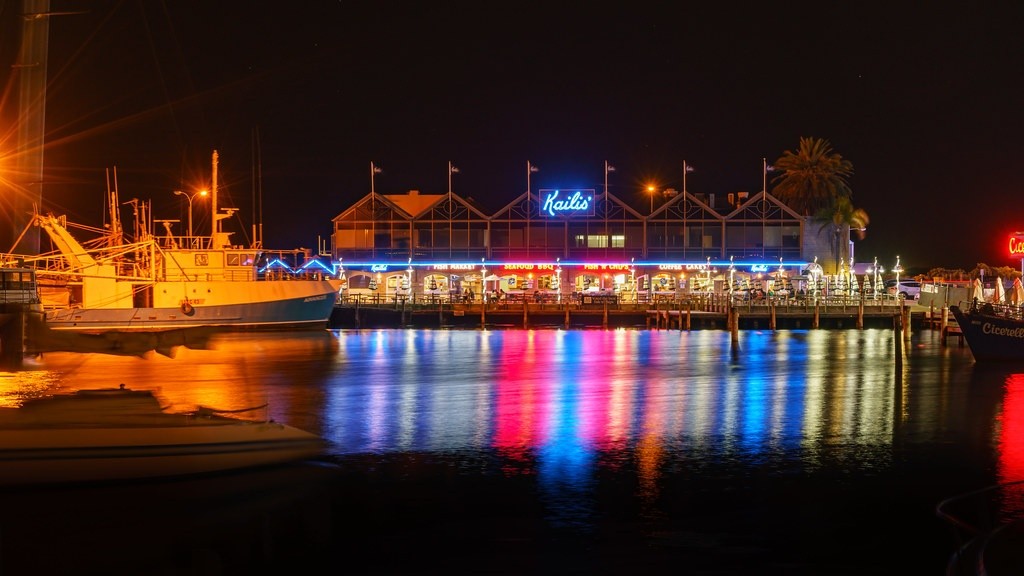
[181,304,194,315]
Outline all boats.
[0,388,338,485]
[12,147,348,336]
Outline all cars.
[886,279,921,300]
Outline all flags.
[685,162,695,173]
[765,159,775,171]
[606,163,617,172]
[530,161,541,174]
[374,165,384,176]
[451,163,464,175]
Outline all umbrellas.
[550,274,557,290]
[583,275,702,291]
[401,277,409,298]
[430,277,437,300]
[481,274,506,291]
[521,277,528,298]
[368,275,378,295]
[342,274,347,292]
[974,276,1024,311]
[449,275,476,289]
[722,274,884,297]
[851,276,860,295]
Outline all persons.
[708,278,714,294]
[454,288,628,306]
[742,289,763,302]
[796,289,804,298]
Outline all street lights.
[838,258,858,297]
[810,256,820,298]
[778,258,784,293]
[405,257,414,305]
[629,258,635,294]
[867,257,884,298]
[701,257,717,292]
[554,257,563,302]
[728,255,737,303]
[174,189,207,247]
[481,258,488,304]
[891,255,903,301]
[647,184,655,214]
